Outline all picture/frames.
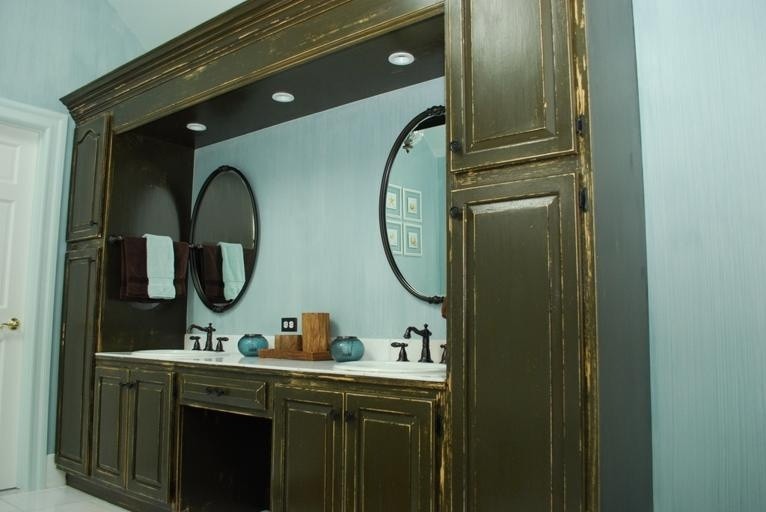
[387,184,424,257]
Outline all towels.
[117,232,189,303]
[197,241,254,304]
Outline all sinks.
[332,362,446,374]
[131,348,230,357]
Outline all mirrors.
[188,164,258,313]
[379,105,445,304]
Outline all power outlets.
[280,317,298,332]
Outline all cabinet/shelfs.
[443,0,655,511]
[51,68,192,481]
[90,367,271,512]
[272,383,441,512]
[179,371,269,412]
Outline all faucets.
[405,323,434,363]
[186,324,216,349]
